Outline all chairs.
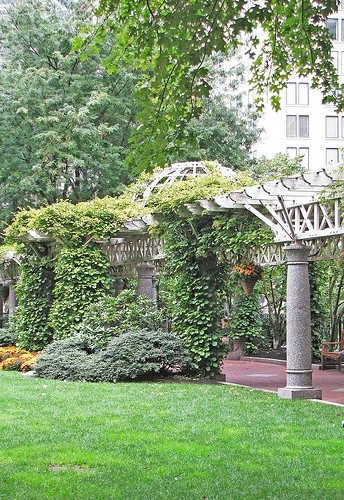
[321,329,344,370]
